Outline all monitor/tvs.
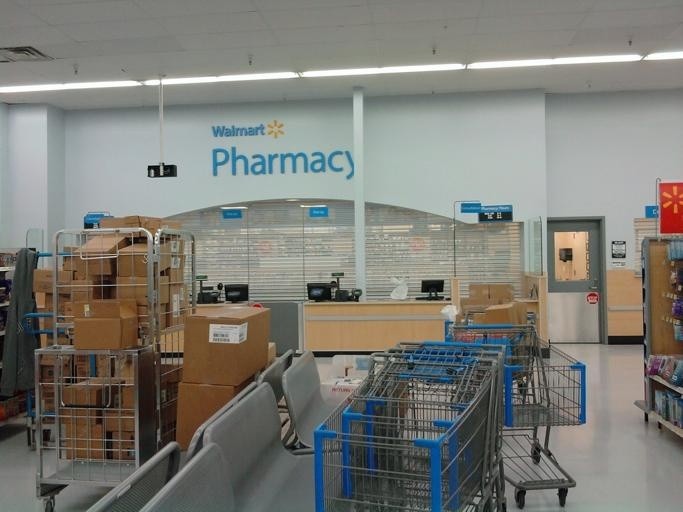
[307,283,331,301]
[224,284,248,303]
[422,280,444,300]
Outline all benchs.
[258,347,295,407]
[200,379,366,512]
[138,439,238,512]
[283,350,364,454]
[80,428,180,512]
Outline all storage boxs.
[31,216,189,459]
[181,306,272,385]
[453,280,531,379]
[175,381,236,452]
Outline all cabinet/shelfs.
[633,228,682,445]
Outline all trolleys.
[314,341,507,511]
[444,319,586,509]
[22,313,65,450]
[35,330,185,511]
